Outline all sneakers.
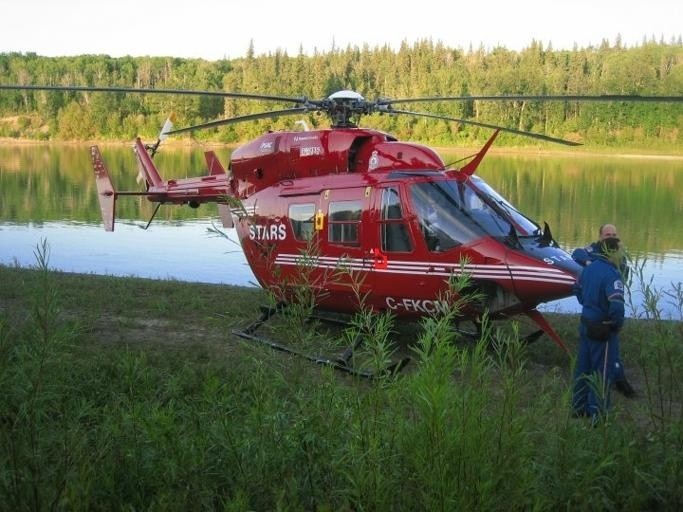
[617,380,638,400]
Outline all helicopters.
[1,85,683,381]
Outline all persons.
[569,222,642,404]
[569,238,628,415]
